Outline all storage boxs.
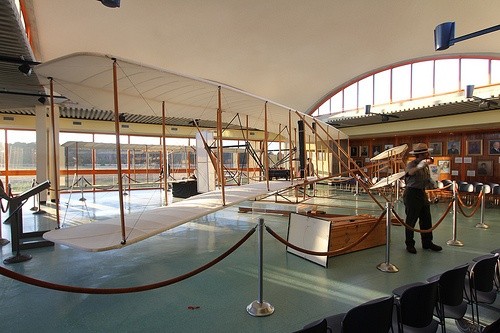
[322,213,388,259]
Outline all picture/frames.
[475,159,494,177]
[446,139,462,157]
[437,159,451,175]
[488,139,500,157]
[356,159,364,168]
[359,145,370,158]
[428,141,443,157]
[466,139,484,157]
[286,211,333,269]
[383,143,394,151]
[372,143,382,158]
[350,146,359,158]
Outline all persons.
[478,162,487,174]
[490,141,500,154]
[159,164,171,190]
[443,162,449,172]
[447,142,459,154]
[403,143,443,253]
[361,147,367,156]
[191,175,197,180]
[469,143,480,154]
[432,166,438,175]
[374,146,379,156]
[306,158,314,190]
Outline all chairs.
[293,248,500,333]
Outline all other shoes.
[422,242,442,251]
[407,246,417,254]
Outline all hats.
[409,143,434,155]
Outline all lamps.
[365,105,399,119]
[37,96,47,105]
[18,63,32,78]
[465,85,495,105]
[432,21,500,51]
